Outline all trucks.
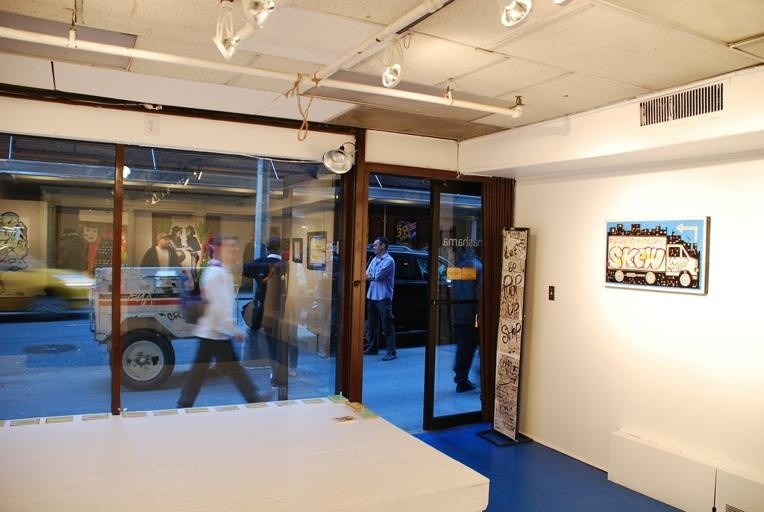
[607,235,700,288]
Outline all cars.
[0,252,95,310]
[366,245,455,348]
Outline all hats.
[170,225,182,234]
[157,233,171,242]
[184,226,195,235]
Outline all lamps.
[145,158,202,205]
[554,0,576,8]
[240,0,276,31]
[322,141,358,176]
[497,0,535,29]
[213,0,252,61]
[380,35,404,89]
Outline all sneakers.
[253,367,298,403]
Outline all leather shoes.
[364,346,378,354]
[454,381,478,393]
[381,352,397,362]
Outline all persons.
[263,246,300,377]
[173,236,273,408]
[364,236,397,361]
[138,233,185,277]
[451,244,483,393]
[227,237,282,368]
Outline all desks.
[0,396,490,512]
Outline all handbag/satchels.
[181,296,209,323]
[242,297,261,330]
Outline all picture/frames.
[292,238,302,263]
[307,232,325,270]
[604,217,709,295]
